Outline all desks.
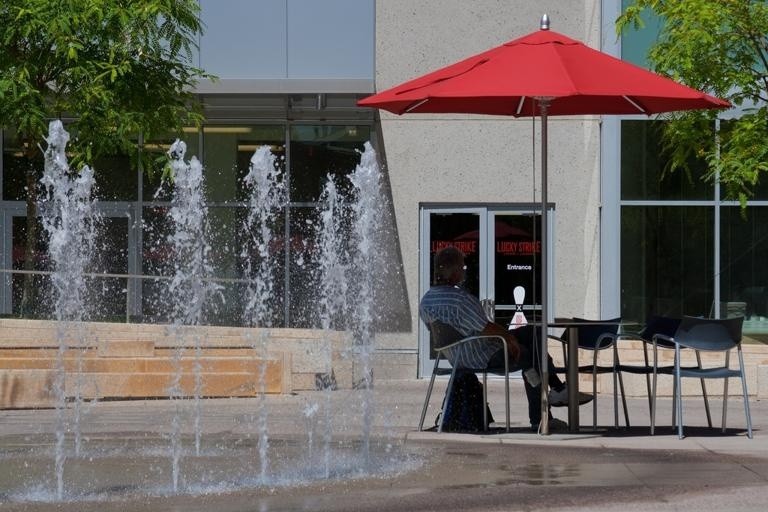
[507,317,638,439]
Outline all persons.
[417,245,594,435]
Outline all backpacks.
[441,370,494,433]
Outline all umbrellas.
[355,9,735,432]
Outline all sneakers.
[548,381,594,406]
[532,418,567,433]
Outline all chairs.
[614,319,713,430]
[538,315,631,433]
[650,316,753,435]
[416,313,541,433]
